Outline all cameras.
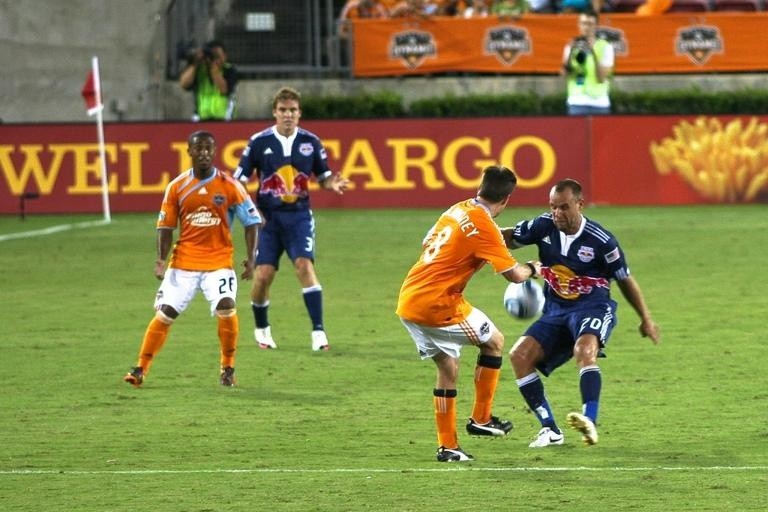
[573,36,588,65]
[202,42,219,62]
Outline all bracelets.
[524,262,536,278]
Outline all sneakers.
[311,330,331,351]
[465,416,515,437]
[528,425,566,449]
[122,367,144,388]
[220,367,237,389]
[253,326,278,349]
[566,412,599,446]
[436,446,475,462]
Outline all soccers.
[503,277,545,319]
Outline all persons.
[559,10,617,118]
[335,0,615,42]
[496,177,659,448]
[232,85,352,353]
[179,39,239,122]
[125,129,261,390]
[395,164,542,461]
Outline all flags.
[80,60,104,117]
